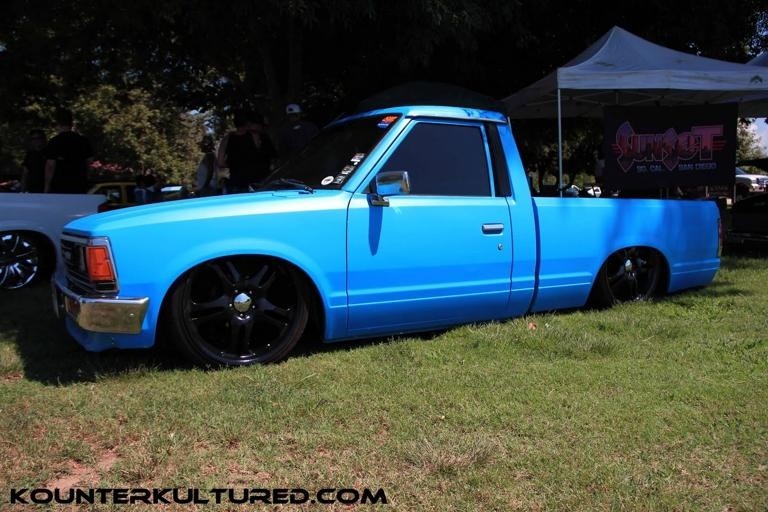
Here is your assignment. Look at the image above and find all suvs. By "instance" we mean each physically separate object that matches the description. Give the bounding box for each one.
[732,165,767,200]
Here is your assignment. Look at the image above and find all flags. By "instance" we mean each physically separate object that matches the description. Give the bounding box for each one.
[597,101,739,190]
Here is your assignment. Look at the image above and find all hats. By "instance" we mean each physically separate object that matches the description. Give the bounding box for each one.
[286,104,300,113]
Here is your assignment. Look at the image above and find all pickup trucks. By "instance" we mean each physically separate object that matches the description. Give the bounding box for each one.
[0,190,112,295]
[79,181,182,214]
[50,103,726,372]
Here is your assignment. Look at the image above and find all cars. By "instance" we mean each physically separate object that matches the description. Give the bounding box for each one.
[718,189,767,262]
[556,181,582,197]
[580,183,619,199]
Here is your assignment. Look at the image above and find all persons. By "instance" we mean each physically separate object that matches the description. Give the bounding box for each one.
[132,101,319,204]
[18,127,48,194]
[44,106,96,192]
